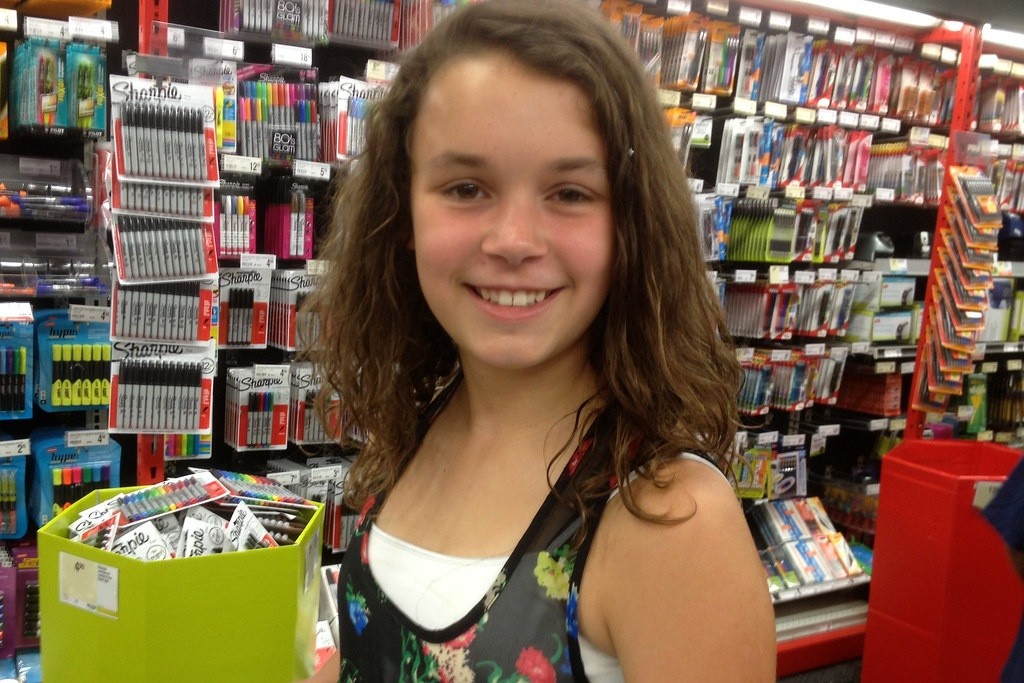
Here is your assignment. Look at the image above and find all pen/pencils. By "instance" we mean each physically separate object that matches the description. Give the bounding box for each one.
[603,0,1024,597]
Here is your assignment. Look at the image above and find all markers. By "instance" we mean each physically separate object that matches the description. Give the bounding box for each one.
[0,0,454,683]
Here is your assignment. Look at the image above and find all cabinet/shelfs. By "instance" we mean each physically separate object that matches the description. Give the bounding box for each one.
[838,259,1024,358]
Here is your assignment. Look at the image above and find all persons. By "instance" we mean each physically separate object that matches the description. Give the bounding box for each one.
[291,0,776,683]
